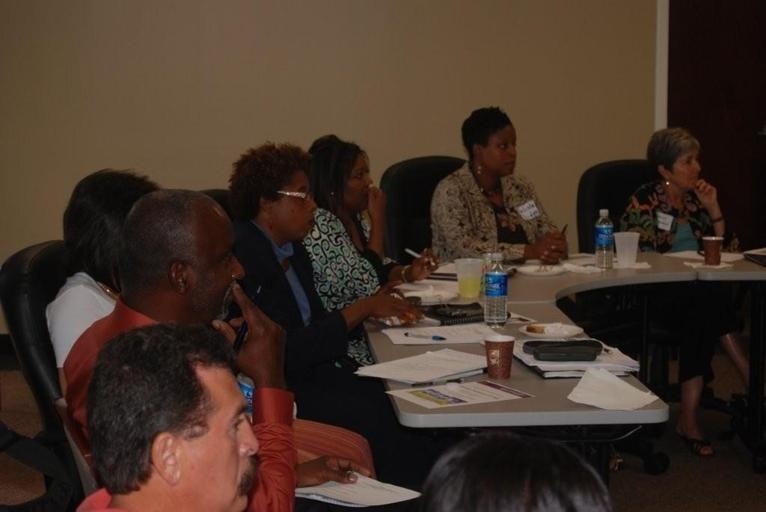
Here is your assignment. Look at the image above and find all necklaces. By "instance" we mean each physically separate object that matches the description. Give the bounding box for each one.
[482,185,502,196]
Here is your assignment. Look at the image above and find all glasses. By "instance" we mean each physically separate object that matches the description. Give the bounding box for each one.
[279,190,313,203]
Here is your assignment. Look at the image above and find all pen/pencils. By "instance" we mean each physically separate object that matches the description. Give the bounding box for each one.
[232,320,247,351]
[404,248,436,266]
[411,379,464,386]
[404,331,446,340]
[561,224,568,234]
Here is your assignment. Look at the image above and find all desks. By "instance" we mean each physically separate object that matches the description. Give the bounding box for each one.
[664,246,766,475]
[357,301,671,483]
[394,244,698,304]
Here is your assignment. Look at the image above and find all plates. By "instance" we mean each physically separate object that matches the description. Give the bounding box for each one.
[518,323,583,339]
[517,264,564,276]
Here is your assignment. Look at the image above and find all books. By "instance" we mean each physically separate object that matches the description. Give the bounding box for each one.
[514,335,640,381]
[352,346,488,388]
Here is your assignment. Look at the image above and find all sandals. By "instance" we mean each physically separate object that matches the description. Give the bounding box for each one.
[680,433,713,457]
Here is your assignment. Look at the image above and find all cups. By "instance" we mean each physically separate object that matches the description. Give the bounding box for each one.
[614,232,641,266]
[484,335,515,380]
[702,236,724,266]
[455,259,484,298]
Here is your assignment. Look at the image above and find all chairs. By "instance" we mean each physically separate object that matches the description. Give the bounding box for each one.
[1,239,99,512]
[577,158,744,475]
[378,154,468,265]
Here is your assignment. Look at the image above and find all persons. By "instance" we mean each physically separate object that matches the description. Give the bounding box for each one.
[422,429,612,512]
[301,135,440,367]
[429,106,571,265]
[228,143,425,428]
[75,320,261,512]
[59,187,374,511]
[44,170,161,371]
[619,125,751,456]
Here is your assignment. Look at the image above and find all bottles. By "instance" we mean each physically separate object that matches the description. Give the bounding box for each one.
[484,252,508,322]
[594,209,614,268]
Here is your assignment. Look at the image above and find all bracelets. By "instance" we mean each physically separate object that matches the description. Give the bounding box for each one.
[711,215,724,224]
